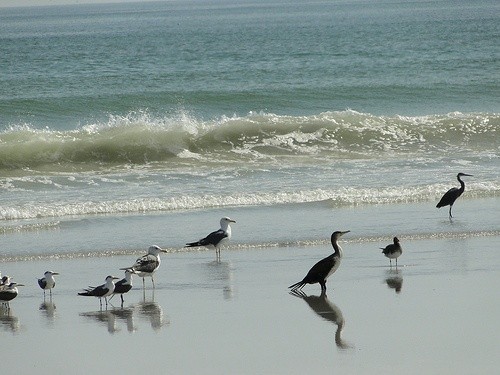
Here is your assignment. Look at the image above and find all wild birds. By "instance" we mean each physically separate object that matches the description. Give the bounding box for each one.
[120,245,168,288]
[38,271,60,294]
[184,217,236,252]
[379,236,403,265]
[0,275,25,314]
[436,172,473,218]
[288,230,351,289]
[78,267,136,308]
